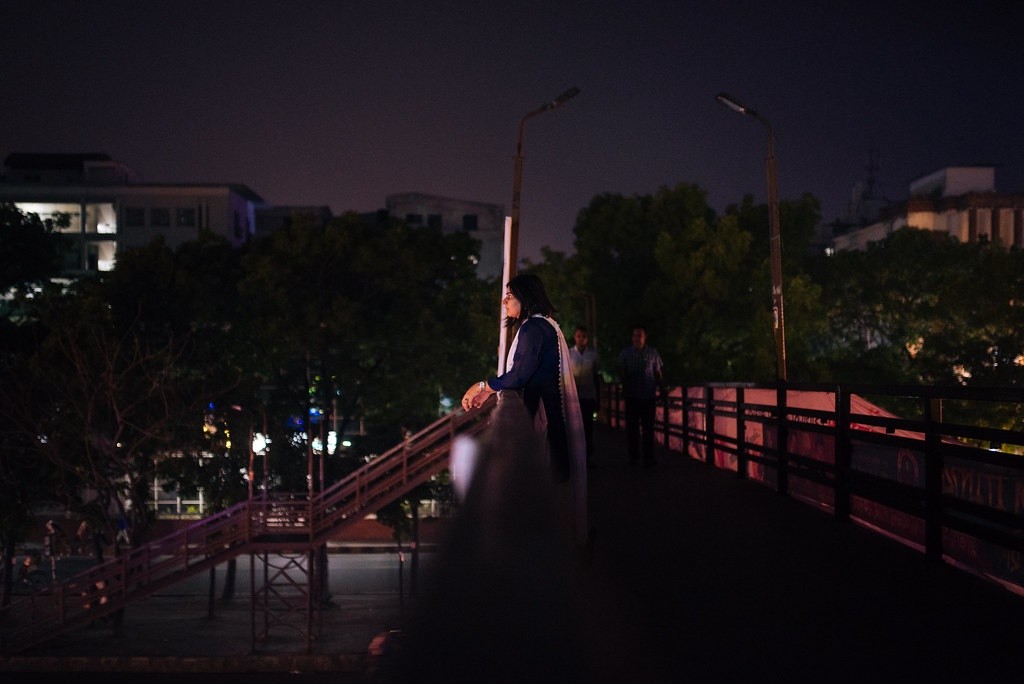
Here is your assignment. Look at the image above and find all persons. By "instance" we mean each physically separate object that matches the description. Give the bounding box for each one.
[459,276,589,634]
[566,326,600,425]
[611,326,666,459]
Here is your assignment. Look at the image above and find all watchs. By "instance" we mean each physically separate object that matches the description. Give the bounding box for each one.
[479,380,486,390]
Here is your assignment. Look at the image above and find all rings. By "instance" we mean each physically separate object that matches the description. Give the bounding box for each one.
[465,399,468,401]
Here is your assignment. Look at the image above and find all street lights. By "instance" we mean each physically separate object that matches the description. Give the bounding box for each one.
[504,83,582,379]
[229,389,460,601]
[712,93,794,494]
